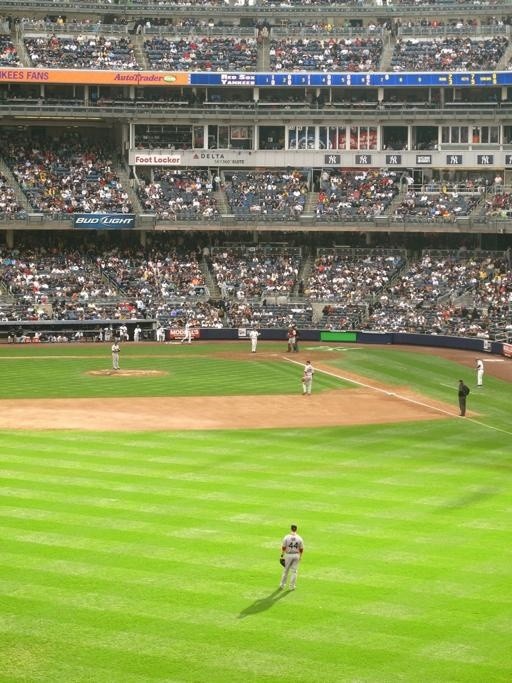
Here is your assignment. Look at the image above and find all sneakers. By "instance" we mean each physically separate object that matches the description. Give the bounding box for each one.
[303,392,312,396]
[279,584,297,591]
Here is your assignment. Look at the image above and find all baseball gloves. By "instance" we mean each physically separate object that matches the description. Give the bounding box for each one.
[280,559,285,566]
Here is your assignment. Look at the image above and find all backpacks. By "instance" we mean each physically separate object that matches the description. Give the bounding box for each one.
[463,385,470,397]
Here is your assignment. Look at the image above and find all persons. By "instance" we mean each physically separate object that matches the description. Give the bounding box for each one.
[303,360,314,395]
[475,356,485,386]
[111,340,121,370]
[0,0,512,74]
[279,525,304,590]
[1,80,511,223]
[458,379,470,416]
[17,228,511,354]
